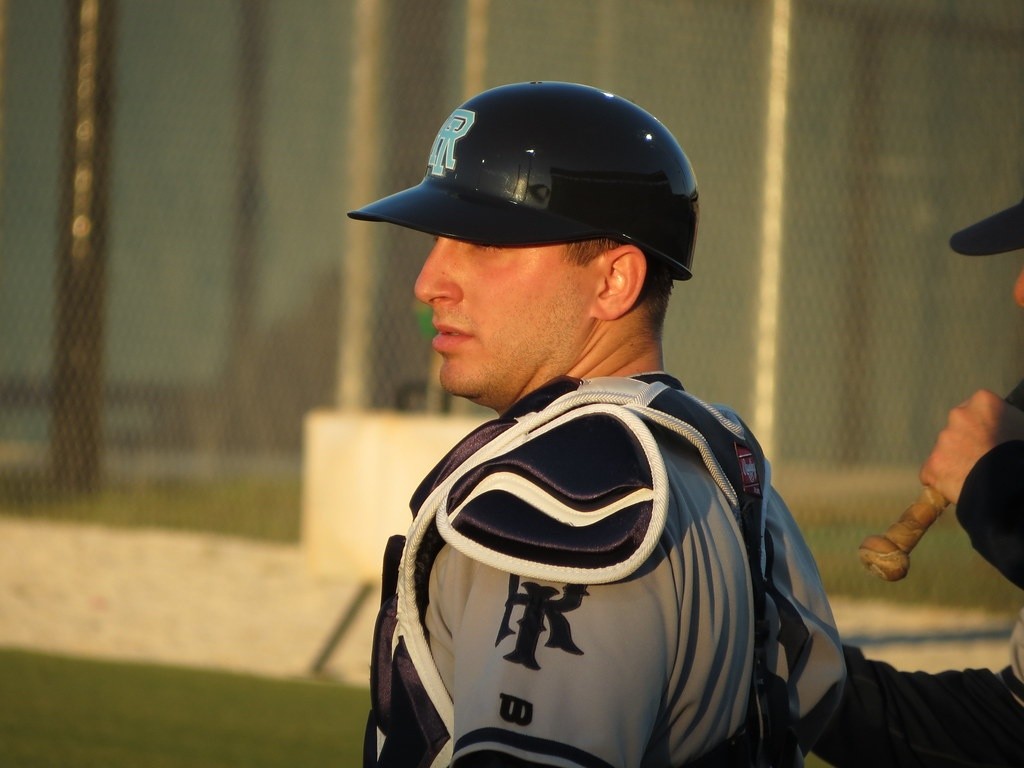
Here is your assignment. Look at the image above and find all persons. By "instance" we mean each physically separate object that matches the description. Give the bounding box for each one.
[347,82,846,768]
[814,200,1024,768]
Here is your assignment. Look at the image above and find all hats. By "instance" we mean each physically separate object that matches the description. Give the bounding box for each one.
[950,204,1024,257]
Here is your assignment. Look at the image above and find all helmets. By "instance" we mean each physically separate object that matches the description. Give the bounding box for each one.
[347,81,695,281]
